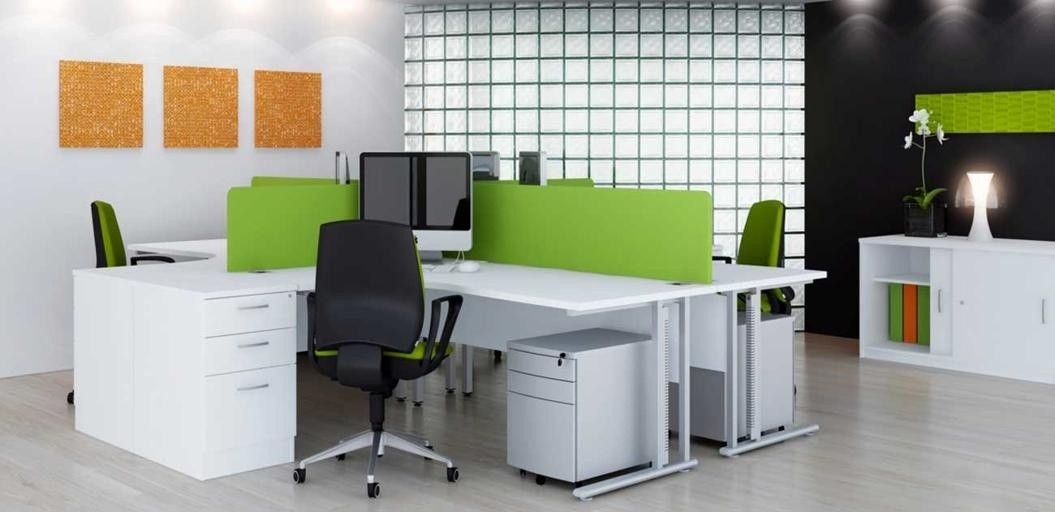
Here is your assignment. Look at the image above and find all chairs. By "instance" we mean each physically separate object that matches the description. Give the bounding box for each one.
[66,200,177,406]
[712,200,797,398]
[291,217,464,494]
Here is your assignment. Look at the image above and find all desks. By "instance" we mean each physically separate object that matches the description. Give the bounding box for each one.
[74,178,830,501]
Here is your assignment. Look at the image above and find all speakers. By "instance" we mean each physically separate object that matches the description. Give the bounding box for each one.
[519,152,540,185]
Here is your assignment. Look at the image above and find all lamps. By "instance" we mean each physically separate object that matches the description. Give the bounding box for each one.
[955,171,999,242]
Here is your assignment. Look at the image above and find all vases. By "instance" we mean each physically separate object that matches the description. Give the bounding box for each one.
[904,203,945,237]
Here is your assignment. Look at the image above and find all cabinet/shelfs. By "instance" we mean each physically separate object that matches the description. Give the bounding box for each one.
[930,238,1055,385]
[860,240,929,366]
[506,326,656,488]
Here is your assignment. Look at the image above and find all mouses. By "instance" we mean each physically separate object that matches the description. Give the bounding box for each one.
[458,262,480,272]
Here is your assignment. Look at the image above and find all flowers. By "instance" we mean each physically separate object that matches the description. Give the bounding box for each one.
[901,109,948,207]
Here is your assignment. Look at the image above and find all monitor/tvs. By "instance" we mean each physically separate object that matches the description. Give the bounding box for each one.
[466,150,500,181]
[358,152,473,260]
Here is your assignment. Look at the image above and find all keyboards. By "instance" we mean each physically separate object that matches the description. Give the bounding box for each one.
[418,264,455,274]
